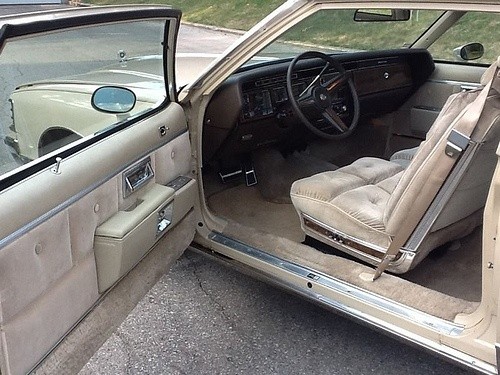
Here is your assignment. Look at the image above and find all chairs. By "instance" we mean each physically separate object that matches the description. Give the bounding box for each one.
[389,140,421,165]
[289,53,500,276]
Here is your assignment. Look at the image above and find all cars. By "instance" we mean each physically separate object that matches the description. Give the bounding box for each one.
[2,1,498,375]
